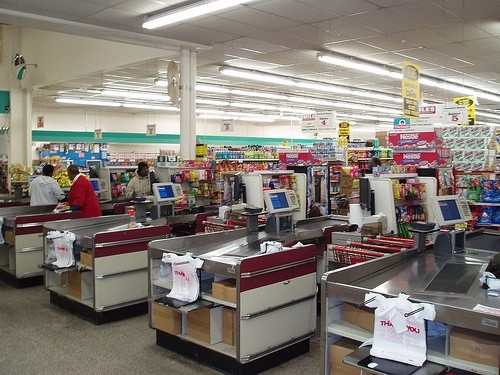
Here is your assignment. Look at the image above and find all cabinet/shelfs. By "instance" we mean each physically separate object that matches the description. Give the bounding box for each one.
[105,124,500,236]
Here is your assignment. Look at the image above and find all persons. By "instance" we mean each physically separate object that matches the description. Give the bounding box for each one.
[29,164,66,206]
[370,156,381,167]
[125,162,153,212]
[59,164,102,218]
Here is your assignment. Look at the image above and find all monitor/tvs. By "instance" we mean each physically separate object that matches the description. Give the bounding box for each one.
[270,191,289,209]
[233,173,247,204]
[91,181,99,192]
[158,185,175,198]
[359,178,376,216]
[438,199,461,221]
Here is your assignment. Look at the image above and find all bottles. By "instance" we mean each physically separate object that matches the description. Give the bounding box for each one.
[128,209,136,229]
[216,151,264,171]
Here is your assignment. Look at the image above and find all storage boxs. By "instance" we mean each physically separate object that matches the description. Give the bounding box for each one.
[5,229,500,375]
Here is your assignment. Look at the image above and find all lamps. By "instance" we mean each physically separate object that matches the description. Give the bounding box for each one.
[53,0,499,125]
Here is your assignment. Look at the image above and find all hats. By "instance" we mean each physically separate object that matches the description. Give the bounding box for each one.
[137,162,149,172]
